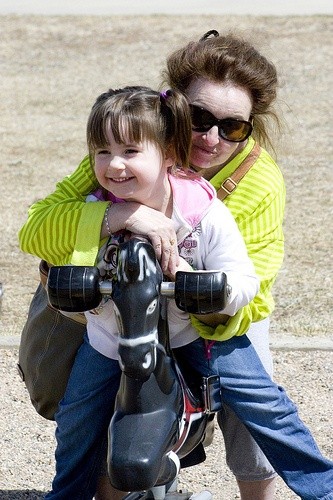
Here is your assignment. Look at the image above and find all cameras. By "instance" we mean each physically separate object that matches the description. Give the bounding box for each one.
[200,375,222,414]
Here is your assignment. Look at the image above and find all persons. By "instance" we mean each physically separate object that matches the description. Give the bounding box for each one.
[17,29,287,500]
[53,85,333,500]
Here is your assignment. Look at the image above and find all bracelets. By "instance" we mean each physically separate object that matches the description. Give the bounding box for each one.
[105,201,114,236]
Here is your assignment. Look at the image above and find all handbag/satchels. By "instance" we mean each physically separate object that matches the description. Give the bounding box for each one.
[15,259,88,421]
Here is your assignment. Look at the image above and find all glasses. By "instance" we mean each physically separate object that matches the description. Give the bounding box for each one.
[188,103,255,143]
